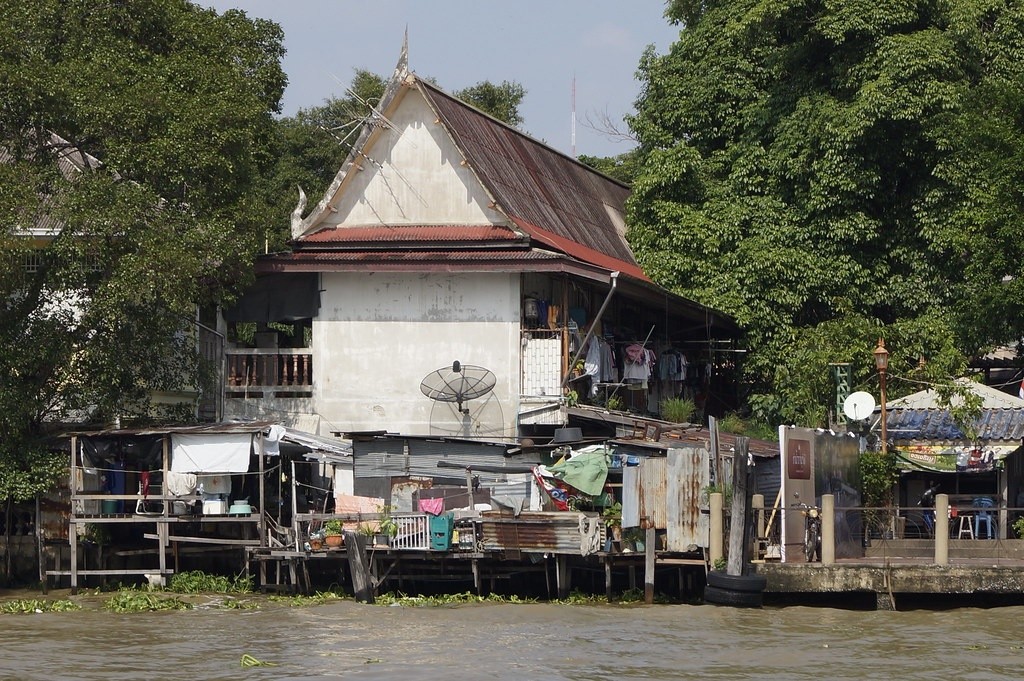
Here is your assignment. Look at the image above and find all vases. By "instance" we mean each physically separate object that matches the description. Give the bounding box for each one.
[309,539,322,550]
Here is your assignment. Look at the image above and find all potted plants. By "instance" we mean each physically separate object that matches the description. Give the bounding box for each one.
[624,527,646,552]
[375,504,399,545]
[324,517,344,548]
[361,520,379,545]
[603,502,623,540]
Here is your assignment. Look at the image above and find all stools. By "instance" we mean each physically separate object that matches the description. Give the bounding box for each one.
[957,516,974,540]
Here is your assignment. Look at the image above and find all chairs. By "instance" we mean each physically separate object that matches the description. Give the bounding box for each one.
[972,497,996,538]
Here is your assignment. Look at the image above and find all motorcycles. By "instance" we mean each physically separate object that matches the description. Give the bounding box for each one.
[790,492,823,562]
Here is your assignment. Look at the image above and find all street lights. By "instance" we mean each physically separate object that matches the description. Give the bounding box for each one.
[873,336,893,538]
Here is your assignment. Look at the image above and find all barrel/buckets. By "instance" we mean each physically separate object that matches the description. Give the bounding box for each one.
[103,499,118,514]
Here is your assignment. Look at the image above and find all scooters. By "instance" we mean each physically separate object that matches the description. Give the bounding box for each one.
[918,484,953,537]
